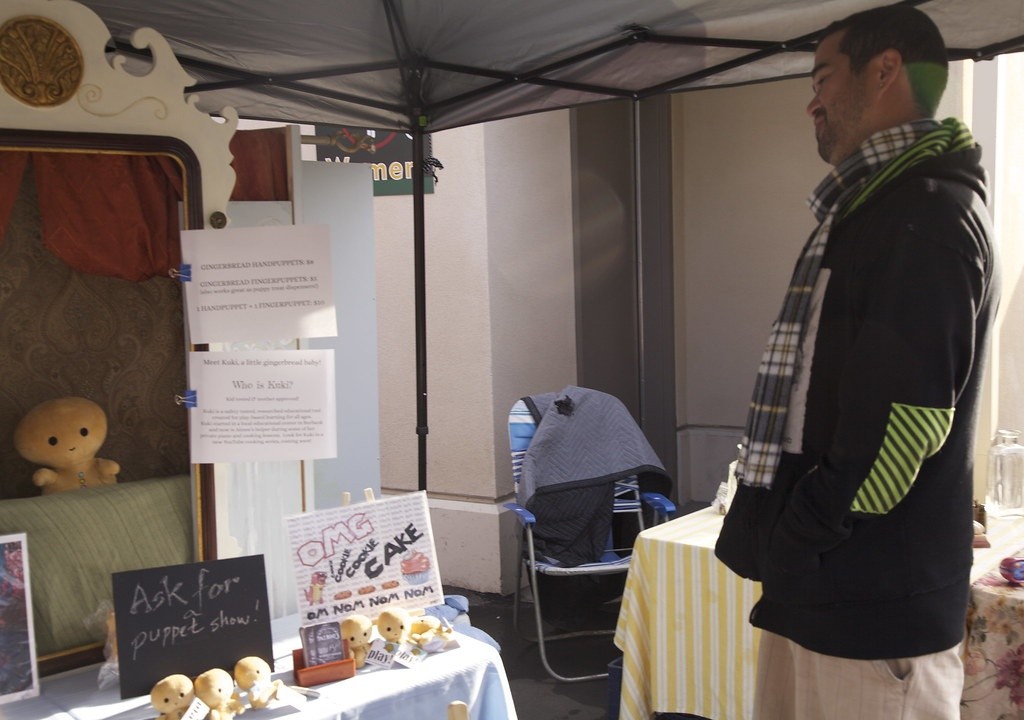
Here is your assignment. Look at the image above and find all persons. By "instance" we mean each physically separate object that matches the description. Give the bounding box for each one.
[712,4,1004,720]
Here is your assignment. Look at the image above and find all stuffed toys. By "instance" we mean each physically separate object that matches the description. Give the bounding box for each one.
[339,614,379,670]
[14,397,122,497]
[233,656,284,711]
[377,605,411,663]
[150,674,204,719]
[193,668,246,720]
[406,612,453,654]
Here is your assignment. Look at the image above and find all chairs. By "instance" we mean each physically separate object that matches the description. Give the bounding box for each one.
[501,386,676,682]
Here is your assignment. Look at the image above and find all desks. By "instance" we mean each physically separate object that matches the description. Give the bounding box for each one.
[0,606,500,720]
[639,506,1024,610]
